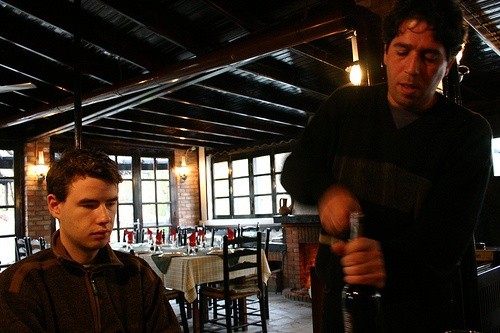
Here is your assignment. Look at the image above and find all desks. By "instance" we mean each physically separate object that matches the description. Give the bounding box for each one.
[135,248,271,333]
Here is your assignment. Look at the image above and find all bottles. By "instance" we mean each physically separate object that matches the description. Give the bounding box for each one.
[342,212,381,333]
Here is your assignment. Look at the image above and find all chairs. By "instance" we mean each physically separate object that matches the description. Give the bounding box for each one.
[14,236,46,262]
[123,223,272,333]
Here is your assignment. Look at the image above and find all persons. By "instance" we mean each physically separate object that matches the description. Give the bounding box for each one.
[0,147,184,333]
[281,0,492,333]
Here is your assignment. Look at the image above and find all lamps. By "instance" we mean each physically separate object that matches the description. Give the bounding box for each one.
[350,38,362,86]
[25,150,51,184]
[171,155,195,181]
